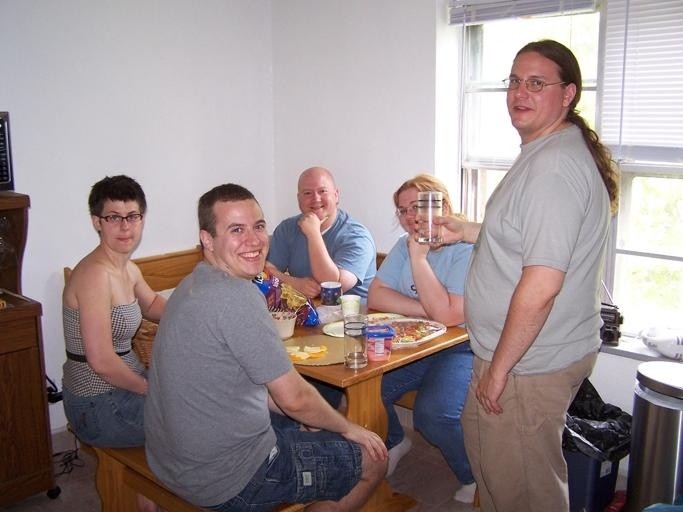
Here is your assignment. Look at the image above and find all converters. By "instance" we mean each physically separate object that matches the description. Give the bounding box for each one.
[48,391,63,403]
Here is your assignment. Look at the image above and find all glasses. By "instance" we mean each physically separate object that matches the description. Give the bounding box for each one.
[502,77,567,93]
[394,204,419,217]
[99,214,143,223]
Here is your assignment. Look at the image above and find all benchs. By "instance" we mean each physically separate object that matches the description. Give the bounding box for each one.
[61,240,205,301]
[65,414,432,512]
[374,252,421,410]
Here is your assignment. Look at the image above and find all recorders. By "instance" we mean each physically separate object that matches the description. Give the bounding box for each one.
[600,279,624,345]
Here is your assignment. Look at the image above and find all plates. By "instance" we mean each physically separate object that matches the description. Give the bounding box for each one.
[322,313,446,348]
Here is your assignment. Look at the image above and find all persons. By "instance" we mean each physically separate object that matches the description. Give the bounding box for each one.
[264,168,378,411]
[60,175,168,449]
[411,39,620,510]
[144,181,389,512]
[367,175,477,503]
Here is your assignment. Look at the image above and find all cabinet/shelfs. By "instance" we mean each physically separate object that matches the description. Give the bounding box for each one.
[0,188,62,511]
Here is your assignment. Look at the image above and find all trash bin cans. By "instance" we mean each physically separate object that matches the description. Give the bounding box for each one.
[562,397,632,512]
[624,360,683,512]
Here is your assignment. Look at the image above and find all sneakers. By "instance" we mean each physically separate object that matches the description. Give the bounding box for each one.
[450,481,479,505]
[384,438,412,479]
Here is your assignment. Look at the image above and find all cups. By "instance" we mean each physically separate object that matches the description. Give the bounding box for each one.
[340,295,363,316]
[342,313,368,368]
[319,283,342,306]
[417,191,443,245]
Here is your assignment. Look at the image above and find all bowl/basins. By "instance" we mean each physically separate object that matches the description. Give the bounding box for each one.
[268,312,295,339]
